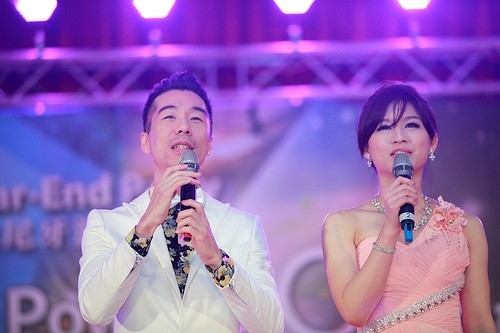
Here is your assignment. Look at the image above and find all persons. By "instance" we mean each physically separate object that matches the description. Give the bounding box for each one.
[320,80,498,333]
[78,73,284,333]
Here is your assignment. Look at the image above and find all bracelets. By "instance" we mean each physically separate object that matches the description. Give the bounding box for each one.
[372,243,397,254]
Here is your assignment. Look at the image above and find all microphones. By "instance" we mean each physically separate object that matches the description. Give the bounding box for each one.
[179,150,200,242]
[392,153,414,242]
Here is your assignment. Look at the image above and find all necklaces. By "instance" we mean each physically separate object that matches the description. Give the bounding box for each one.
[371,194,432,231]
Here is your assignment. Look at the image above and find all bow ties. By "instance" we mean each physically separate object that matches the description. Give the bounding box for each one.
[169,188,205,208]
